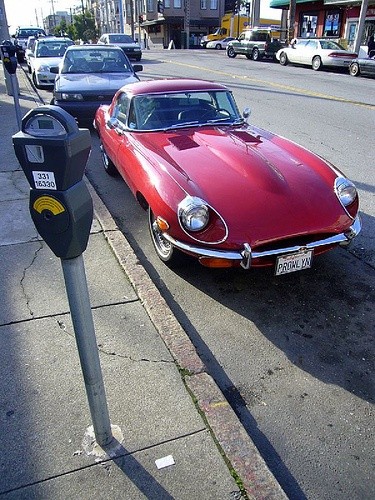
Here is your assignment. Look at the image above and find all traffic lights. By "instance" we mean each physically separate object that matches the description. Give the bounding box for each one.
[139,16,143,23]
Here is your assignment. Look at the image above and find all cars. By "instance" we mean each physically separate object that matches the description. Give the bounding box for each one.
[97,33,142,61]
[29,38,75,88]
[50,45,142,124]
[206,37,235,49]
[24,35,56,73]
[275,39,357,71]
[349,50,375,77]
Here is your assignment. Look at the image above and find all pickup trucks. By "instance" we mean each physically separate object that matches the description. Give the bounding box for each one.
[226,30,283,61]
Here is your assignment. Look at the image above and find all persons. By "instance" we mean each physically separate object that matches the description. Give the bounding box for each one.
[10,35,16,45]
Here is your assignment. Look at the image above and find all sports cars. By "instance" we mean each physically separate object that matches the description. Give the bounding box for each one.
[93,79,361,276]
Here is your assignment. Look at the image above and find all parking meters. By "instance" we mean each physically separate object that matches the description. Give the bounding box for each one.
[0,39,23,131]
[11,106,112,446]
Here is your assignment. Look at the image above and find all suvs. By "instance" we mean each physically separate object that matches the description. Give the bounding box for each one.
[12,28,47,64]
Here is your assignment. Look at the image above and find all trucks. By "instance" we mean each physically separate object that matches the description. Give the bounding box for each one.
[200,15,293,48]
